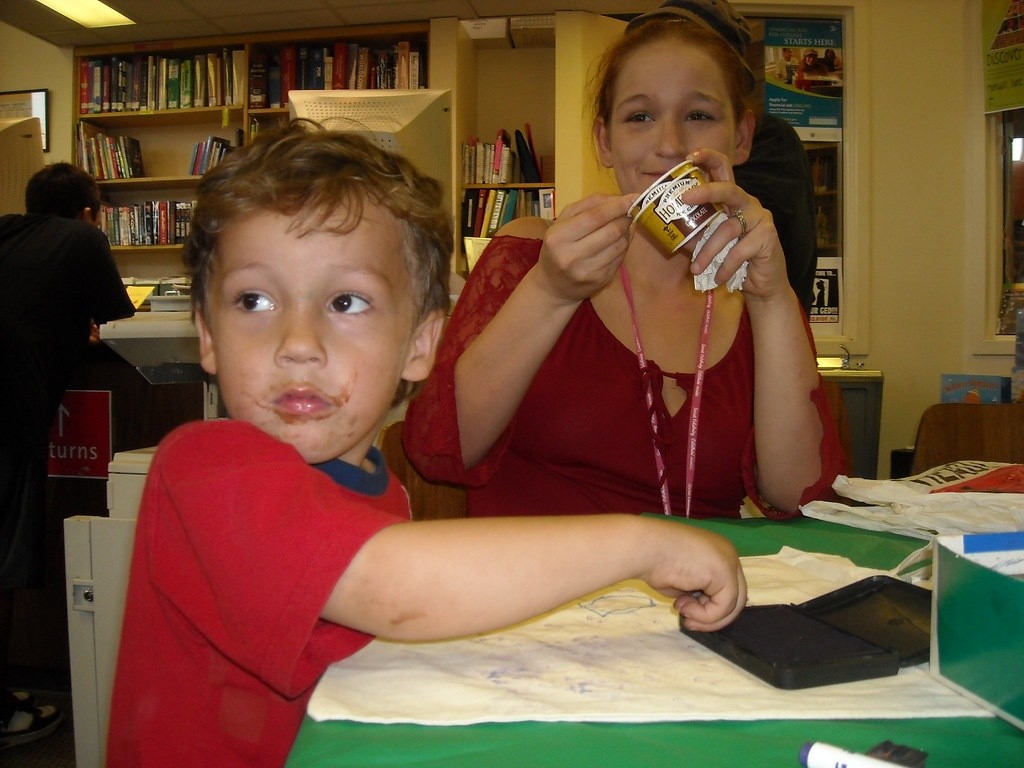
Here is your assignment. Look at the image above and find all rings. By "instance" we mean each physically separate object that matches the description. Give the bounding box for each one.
[729,207,748,238]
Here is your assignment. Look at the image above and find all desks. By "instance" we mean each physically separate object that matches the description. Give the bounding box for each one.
[275,515,1022,768]
[814,368,884,481]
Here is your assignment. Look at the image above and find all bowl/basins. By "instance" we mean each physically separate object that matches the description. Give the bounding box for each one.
[627,160,727,256]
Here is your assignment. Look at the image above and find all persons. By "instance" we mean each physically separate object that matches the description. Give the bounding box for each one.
[403,1,856,523]
[822,48,842,71]
[795,47,833,94]
[108,112,747,768]
[774,45,801,84]
[627,1,819,330]
[1,161,136,750]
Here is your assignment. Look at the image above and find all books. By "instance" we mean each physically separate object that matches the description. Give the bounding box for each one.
[74,119,146,181]
[247,39,428,110]
[79,46,246,115]
[95,199,199,246]
[188,116,262,178]
[461,119,555,273]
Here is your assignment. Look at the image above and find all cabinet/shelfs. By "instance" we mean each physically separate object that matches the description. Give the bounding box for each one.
[63,15,557,315]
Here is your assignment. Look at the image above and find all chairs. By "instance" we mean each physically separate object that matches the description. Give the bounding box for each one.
[908,403,1023,478]
